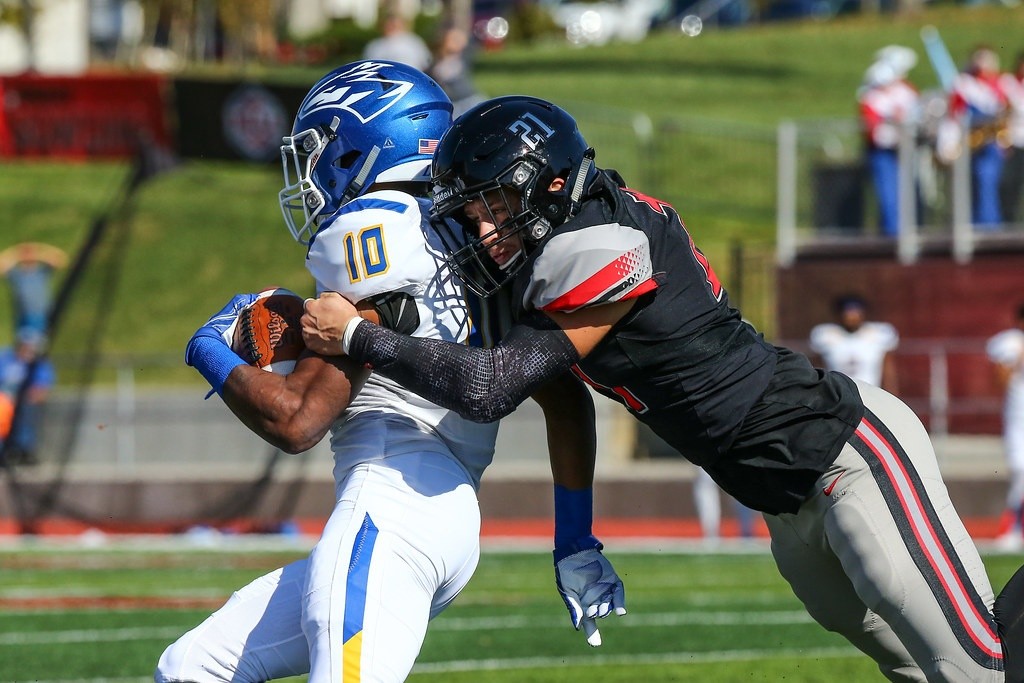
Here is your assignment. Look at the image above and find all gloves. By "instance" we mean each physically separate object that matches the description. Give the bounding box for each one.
[553,536,626,645]
[185,293,260,365]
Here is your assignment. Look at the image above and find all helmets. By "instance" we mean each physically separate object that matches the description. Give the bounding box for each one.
[279,60,453,246]
[428,95,596,299]
[833,290,869,315]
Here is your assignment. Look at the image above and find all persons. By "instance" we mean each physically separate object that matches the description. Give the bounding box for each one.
[300,97,1005,683]
[155,59,627,683]
[808,291,898,397]
[857,44,1024,234]
[988,302,1024,547]
[0,243,72,466]
[153,0,475,98]
[992,563,1024,683]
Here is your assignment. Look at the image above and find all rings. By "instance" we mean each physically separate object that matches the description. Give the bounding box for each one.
[303,298,314,309]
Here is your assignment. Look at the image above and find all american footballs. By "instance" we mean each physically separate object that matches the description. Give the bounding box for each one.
[232,285,305,375]
